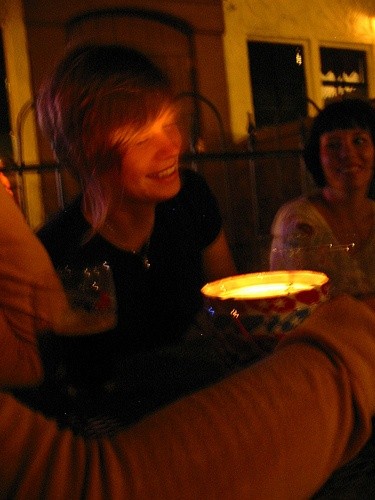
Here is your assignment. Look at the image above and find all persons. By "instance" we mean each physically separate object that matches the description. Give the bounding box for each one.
[33,33,238,334]
[0,293,375,499]
[2,161,72,391]
[269,91,374,310]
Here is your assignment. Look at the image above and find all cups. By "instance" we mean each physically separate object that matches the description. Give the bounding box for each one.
[29,266,120,438]
[201,269,332,383]
